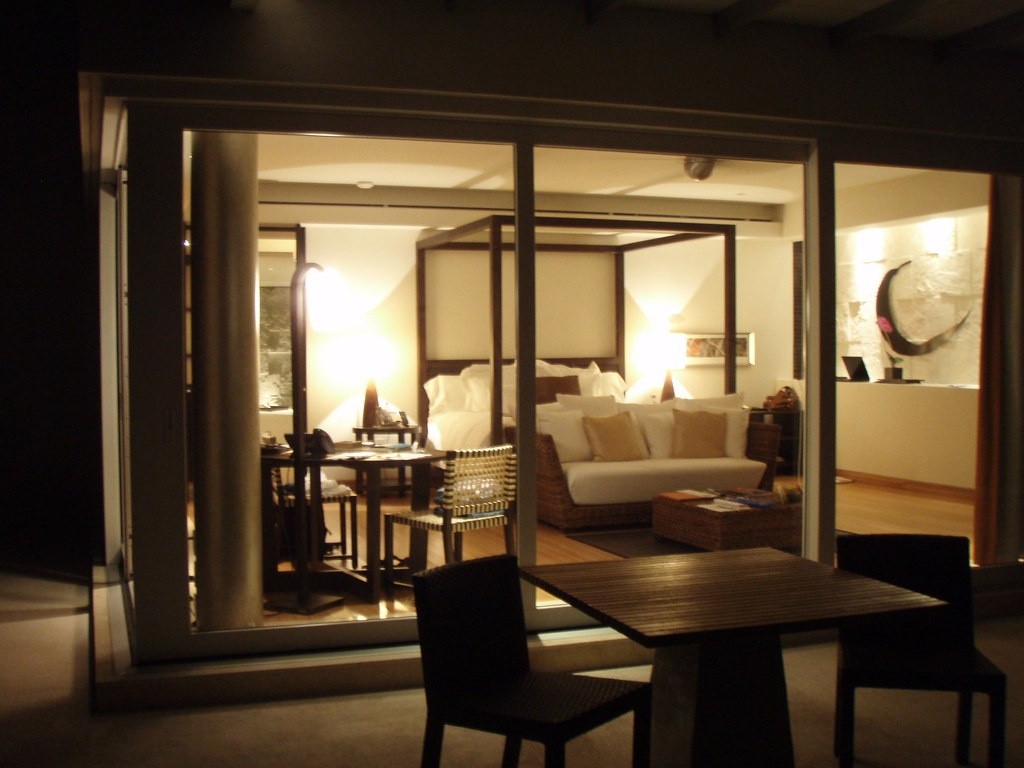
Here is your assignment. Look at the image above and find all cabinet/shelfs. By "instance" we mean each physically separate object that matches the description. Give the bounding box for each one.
[751,409,801,475]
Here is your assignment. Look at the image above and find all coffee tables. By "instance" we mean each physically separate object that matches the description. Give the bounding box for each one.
[654,487,802,553]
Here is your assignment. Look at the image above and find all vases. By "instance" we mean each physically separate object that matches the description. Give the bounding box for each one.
[884,366,902,380]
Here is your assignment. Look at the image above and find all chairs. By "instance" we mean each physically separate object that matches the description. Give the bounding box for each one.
[412,552,654,768]
[386,443,516,598]
[835,534,1007,768]
[268,438,359,569]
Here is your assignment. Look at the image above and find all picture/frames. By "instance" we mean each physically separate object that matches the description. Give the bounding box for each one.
[683,332,755,366]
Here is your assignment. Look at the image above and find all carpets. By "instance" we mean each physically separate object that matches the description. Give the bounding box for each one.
[566,529,853,560]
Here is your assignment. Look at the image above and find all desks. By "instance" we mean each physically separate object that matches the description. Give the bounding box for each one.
[261,442,449,604]
[353,427,423,500]
[519,545,949,768]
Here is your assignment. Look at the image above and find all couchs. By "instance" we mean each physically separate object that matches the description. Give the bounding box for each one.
[505,401,780,530]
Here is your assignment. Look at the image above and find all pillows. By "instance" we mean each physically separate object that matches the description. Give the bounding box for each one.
[423,358,626,414]
[538,391,752,463]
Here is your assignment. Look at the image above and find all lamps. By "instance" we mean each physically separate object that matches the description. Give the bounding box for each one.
[685,156,717,182]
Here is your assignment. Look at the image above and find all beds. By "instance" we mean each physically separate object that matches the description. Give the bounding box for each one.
[421,358,715,507]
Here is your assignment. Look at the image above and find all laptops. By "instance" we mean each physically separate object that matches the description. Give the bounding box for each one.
[842,356,870,382]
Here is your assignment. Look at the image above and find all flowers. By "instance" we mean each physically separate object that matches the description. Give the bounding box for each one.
[876,317,904,367]
